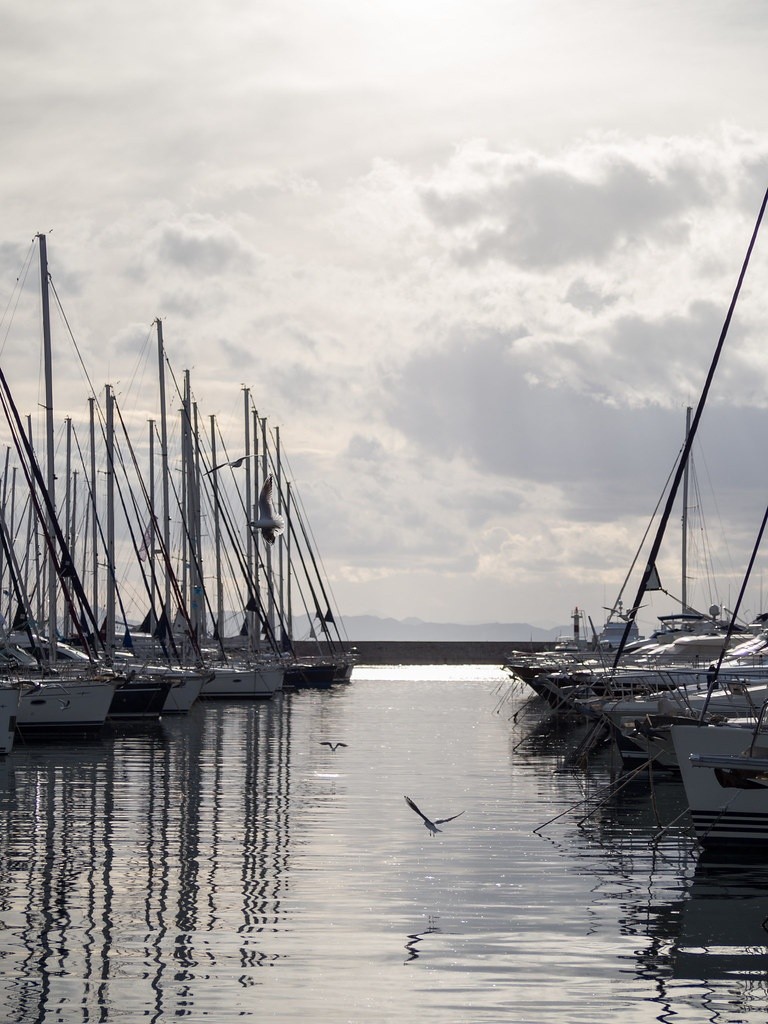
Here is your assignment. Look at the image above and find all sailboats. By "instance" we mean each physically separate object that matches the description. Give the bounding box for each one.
[0,222,358,756]
[501,402,768,855]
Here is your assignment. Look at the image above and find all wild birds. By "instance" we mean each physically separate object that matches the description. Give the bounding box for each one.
[201,454,263,477]
[319,741,349,755]
[403,795,465,837]
[244,472,285,547]
